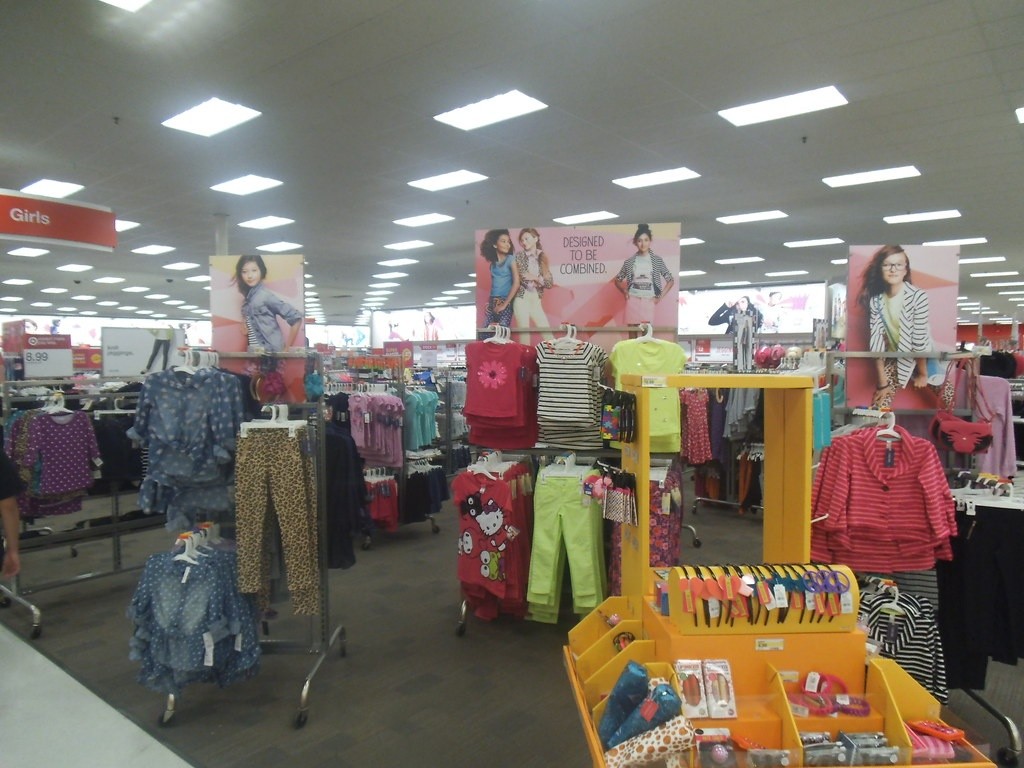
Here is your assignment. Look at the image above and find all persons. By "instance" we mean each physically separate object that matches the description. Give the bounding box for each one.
[0,446,23,579]
[614,223,675,339]
[140,328,173,374]
[423,312,439,340]
[759,291,784,333]
[389,322,410,341]
[343,333,354,346]
[168,321,205,346]
[477,229,521,341]
[854,245,955,410]
[513,228,555,346]
[229,255,303,377]
[25,318,61,335]
[708,296,764,335]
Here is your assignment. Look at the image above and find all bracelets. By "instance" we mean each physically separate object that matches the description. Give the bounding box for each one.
[786,673,869,718]
[876,381,890,390]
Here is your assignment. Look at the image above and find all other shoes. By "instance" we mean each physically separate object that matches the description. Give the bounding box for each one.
[141,368,150,374]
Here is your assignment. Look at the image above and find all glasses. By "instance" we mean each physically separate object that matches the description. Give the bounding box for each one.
[881,262,907,270]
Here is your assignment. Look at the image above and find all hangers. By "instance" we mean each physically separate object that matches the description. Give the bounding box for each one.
[831,408,900,445]
[737,442,764,462]
[775,351,845,394]
[864,575,906,615]
[35,350,308,566]
[949,471,1024,516]
[324,380,442,484]
[467,323,669,489]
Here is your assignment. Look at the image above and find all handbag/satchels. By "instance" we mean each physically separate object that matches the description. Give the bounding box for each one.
[928,355,996,455]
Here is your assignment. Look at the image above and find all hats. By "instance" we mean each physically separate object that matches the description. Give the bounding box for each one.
[753,345,801,367]
